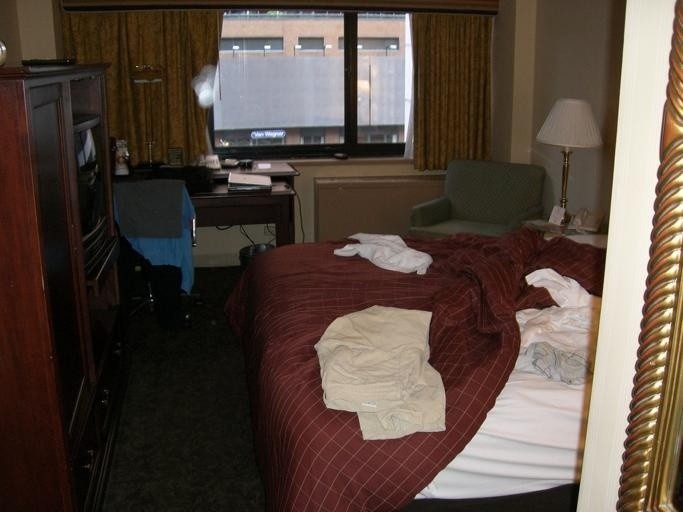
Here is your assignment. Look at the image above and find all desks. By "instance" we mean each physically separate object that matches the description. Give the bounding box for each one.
[113,162,300,247]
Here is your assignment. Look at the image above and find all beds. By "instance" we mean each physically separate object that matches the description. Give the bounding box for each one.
[248,233,607,510]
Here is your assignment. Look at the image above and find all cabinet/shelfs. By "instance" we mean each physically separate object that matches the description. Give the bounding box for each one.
[0,62,131,509]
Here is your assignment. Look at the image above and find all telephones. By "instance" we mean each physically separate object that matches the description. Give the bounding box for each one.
[573,207,606,232]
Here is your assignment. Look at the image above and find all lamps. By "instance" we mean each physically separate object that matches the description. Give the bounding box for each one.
[536,97,605,228]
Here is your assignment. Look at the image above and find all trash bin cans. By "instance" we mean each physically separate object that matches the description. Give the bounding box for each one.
[240,243,275,272]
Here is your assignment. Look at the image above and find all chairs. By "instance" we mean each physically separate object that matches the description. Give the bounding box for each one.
[114,180,202,320]
[408,160,547,243]
